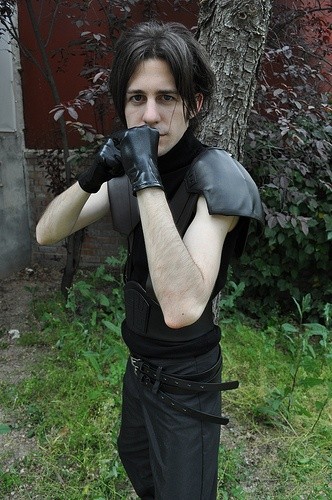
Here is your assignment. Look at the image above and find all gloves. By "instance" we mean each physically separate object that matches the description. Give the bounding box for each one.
[112,124,167,197]
[78,136,125,194]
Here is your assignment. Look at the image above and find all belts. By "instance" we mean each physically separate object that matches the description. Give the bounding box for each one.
[127,343,239,425]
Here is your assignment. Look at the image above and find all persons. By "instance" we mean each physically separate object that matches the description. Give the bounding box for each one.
[35,19,262,500]
